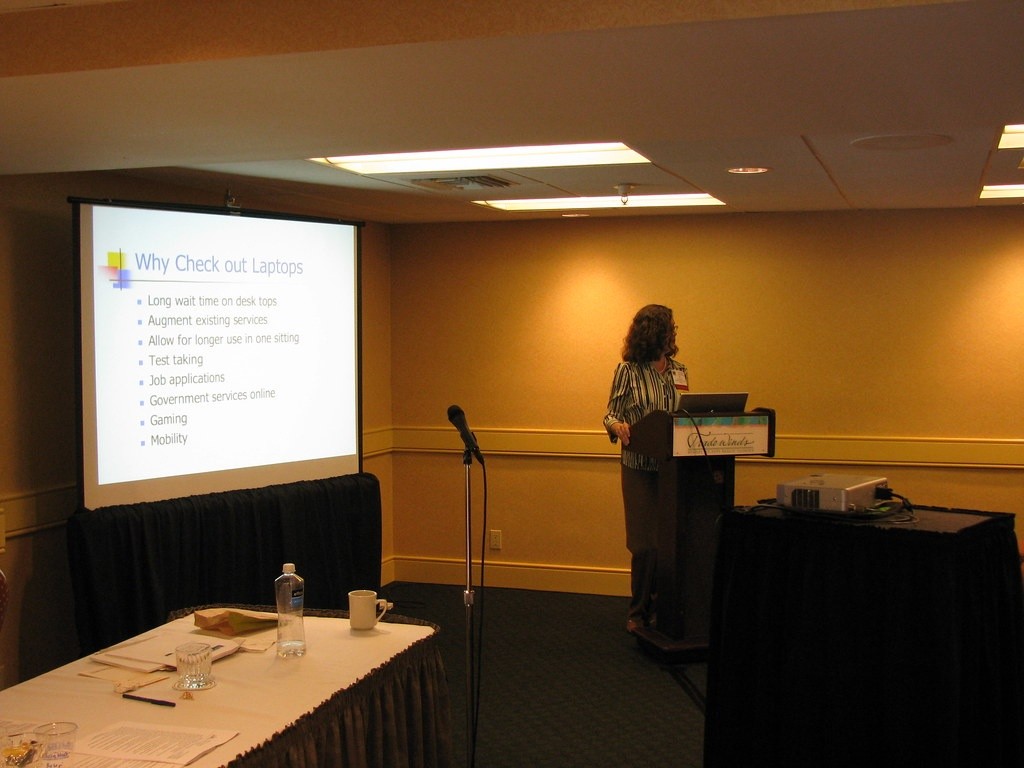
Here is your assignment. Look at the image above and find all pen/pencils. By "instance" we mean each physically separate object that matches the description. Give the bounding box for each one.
[123,693,176,708]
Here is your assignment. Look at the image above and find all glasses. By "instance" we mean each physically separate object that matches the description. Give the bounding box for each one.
[665,325,678,335]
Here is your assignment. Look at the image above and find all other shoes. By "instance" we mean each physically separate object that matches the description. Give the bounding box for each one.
[627,619,636,632]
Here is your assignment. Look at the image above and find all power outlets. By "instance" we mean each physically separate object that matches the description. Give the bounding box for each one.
[490,530,502,549]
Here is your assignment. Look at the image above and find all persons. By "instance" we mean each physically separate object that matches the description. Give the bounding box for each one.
[602,304,689,629]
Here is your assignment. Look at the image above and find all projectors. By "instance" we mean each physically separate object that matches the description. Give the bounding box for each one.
[776,473,888,512]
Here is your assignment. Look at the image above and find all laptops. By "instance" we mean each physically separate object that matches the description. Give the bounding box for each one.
[676,392,750,414]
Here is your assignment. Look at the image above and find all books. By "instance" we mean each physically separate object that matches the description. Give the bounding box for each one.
[90,631,246,673]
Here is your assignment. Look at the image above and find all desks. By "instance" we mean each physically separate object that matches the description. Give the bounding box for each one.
[704,503,1024,768]
[0,602,452,768]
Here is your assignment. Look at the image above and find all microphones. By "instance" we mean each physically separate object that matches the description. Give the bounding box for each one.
[447,405,485,465]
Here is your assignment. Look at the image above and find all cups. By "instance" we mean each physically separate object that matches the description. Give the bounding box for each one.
[33,721,77,768]
[348,590,387,630]
[175,643,212,684]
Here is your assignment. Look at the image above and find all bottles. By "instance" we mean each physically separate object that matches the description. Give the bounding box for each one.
[275,563,305,658]
